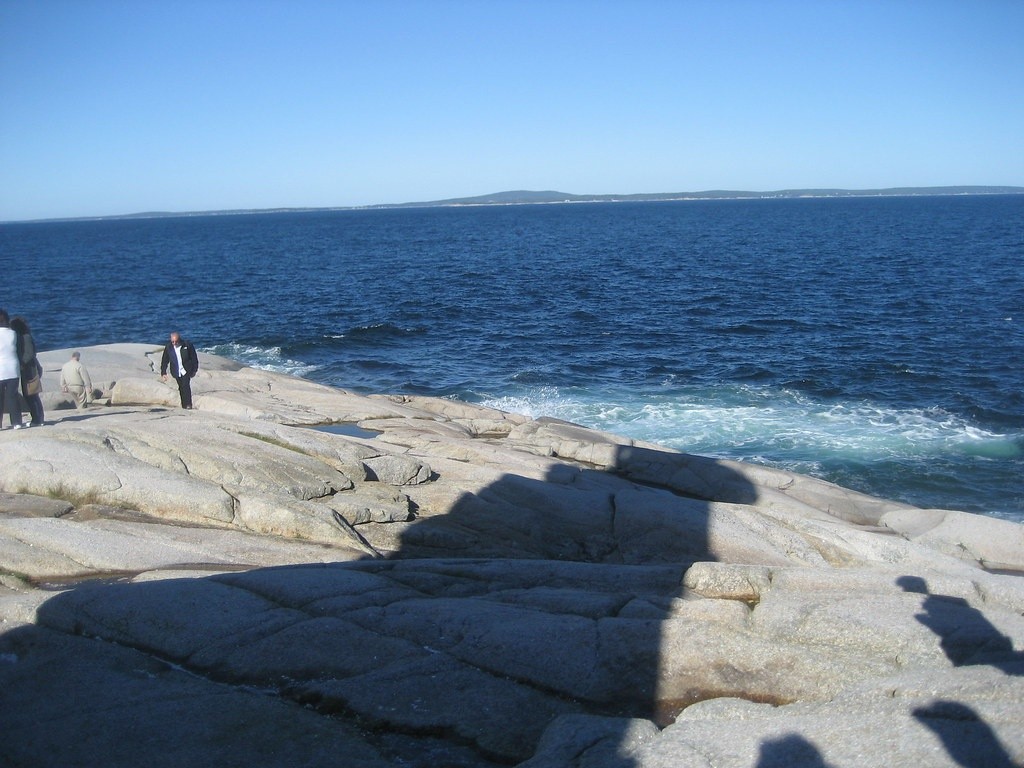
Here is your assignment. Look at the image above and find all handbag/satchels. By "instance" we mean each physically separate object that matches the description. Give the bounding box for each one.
[26,375,42,396]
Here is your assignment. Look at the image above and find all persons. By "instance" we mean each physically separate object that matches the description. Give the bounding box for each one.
[60,352,92,409]
[0,311,23,430]
[161,333,198,410]
[10,318,44,427]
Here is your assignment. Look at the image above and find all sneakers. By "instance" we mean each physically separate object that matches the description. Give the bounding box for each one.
[0,428,2,431]
[26,421,45,428]
[13,425,22,430]
